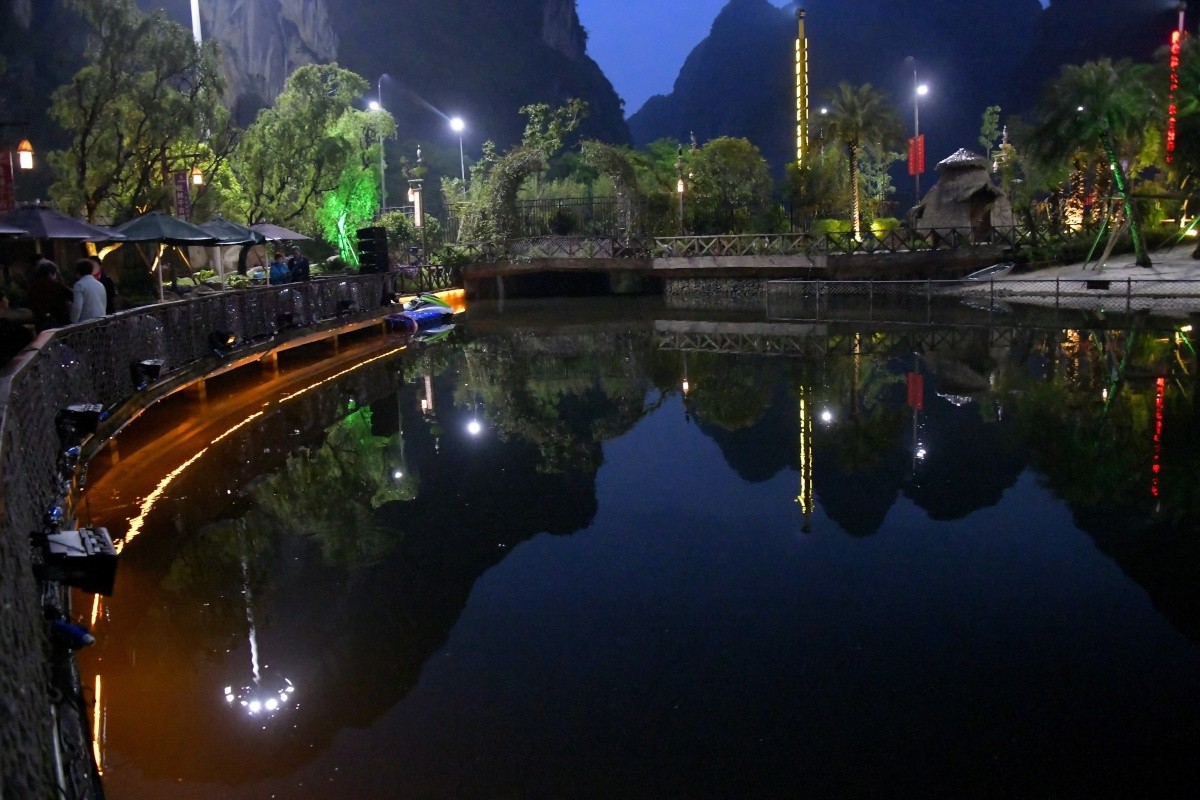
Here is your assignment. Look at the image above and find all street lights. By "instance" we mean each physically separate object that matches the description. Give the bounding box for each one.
[376,73,386,209]
[674,144,689,235]
[450,116,471,200]
[912,70,928,205]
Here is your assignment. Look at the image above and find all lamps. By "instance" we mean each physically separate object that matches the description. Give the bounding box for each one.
[138,358,165,381]
[55,403,105,435]
[343,300,358,311]
[215,328,236,347]
[28,525,117,599]
[283,312,300,324]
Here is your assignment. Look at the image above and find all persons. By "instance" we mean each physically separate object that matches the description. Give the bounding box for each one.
[288,246,310,282]
[29,256,116,337]
[270,254,293,286]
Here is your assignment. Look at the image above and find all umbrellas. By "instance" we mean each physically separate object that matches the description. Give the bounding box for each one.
[194,217,267,296]
[248,223,315,288]
[0,204,128,242]
[81,210,222,303]
